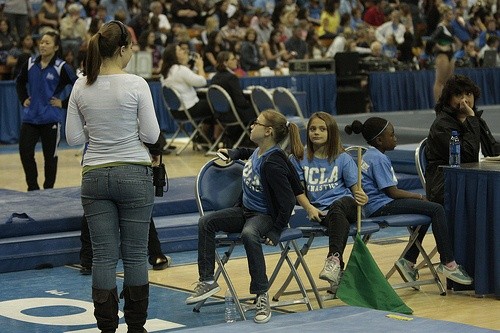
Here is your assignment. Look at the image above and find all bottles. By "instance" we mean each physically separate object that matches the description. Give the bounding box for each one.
[449,131,461,168]
[225,288,237,323]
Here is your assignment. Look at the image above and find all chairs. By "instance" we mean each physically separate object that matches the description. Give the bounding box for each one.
[346,147,446,296]
[250,85,274,117]
[415,138,428,188]
[192,157,312,321]
[161,85,212,155]
[207,84,250,155]
[272,86,307,146]
[272,201,380,308]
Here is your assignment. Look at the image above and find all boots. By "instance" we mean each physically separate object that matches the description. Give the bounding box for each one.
[120,282,150,333]
[91,285,121,333]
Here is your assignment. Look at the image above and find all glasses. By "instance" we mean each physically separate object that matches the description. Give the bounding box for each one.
[252,121,269,128]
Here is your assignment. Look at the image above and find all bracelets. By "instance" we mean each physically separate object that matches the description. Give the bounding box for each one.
[419,193,422,199]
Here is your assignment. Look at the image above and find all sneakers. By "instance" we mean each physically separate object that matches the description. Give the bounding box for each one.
[435,263,474,286]
[394,258,421,289]
[327,270,345,294]
[253,293,272,324]
[318,252,341,283]
[184,281,221,305]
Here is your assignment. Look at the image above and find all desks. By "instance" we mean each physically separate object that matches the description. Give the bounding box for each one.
[0,73,336,142]
[438,160,500,301]
[362,66,500,112]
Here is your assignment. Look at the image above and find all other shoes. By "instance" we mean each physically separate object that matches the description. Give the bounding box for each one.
[80,266,92,275]
[153,255,172,270]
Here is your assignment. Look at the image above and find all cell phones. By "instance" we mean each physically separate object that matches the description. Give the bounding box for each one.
[216,151,229,161]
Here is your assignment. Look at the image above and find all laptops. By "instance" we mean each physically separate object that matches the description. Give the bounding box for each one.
[482,50,497,67]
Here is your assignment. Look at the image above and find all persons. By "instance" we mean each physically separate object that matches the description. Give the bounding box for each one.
[0,0,500,81]
[185,109,325,324]
[344,117,474,290]
[289,111,368,283]
[428,4,457,104]
[211,51,258,147]
[162,42,222,143]
[15,31,77,191]
[79,134,172,276]
[64,20,161,333]
[425,74,500,207]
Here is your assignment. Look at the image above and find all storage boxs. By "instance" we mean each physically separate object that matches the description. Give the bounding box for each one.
[289,59,336,74]
